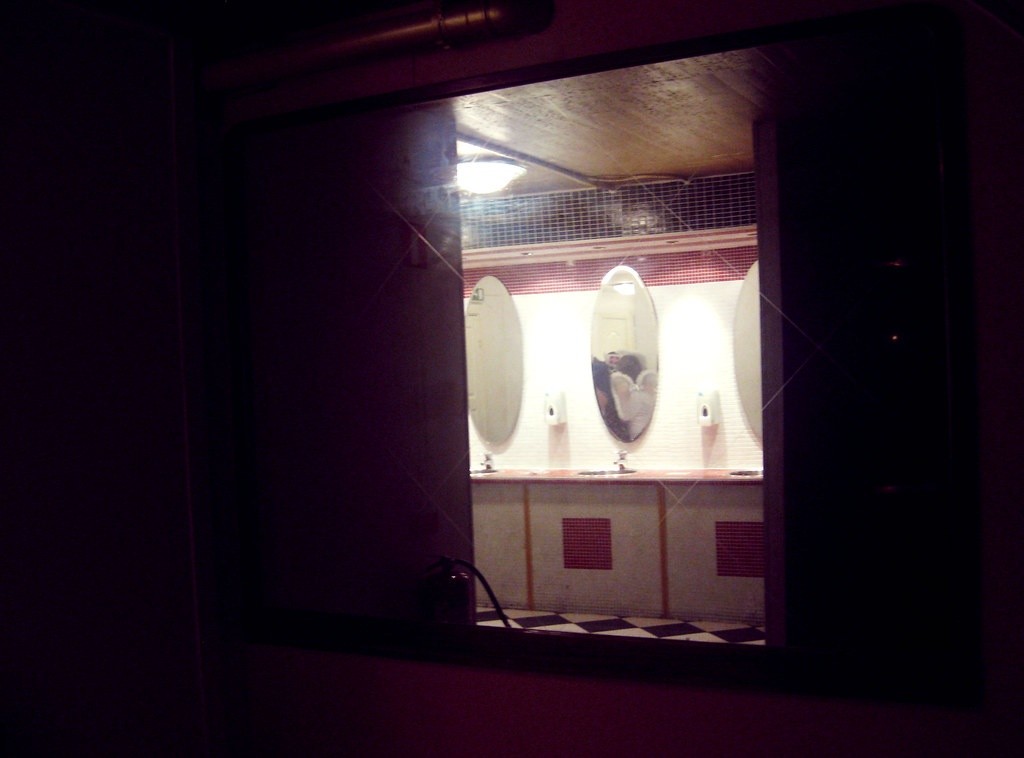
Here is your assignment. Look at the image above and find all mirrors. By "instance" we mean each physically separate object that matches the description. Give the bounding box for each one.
[589,266,660,444]
[464,276,525,446]
[734,261,764,446]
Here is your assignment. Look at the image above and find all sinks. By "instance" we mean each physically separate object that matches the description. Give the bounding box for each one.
[469,469,498,474]
[577,470,639,475]
[729,470,764,475]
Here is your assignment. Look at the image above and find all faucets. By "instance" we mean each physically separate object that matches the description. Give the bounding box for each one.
[613,450,628,471]
[480,451,494,469]
